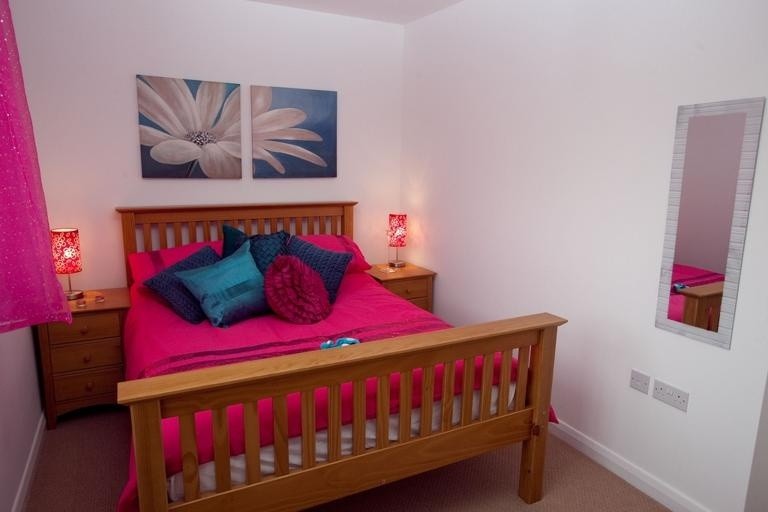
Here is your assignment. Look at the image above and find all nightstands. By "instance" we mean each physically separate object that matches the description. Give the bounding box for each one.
[362,259,437,316]
[36,287,129,428]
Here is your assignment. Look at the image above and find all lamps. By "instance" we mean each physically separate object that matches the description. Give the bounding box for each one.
[48,228,84,301]
[387,213,407,268]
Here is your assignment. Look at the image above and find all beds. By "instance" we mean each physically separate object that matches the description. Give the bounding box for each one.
[112,200,569,512]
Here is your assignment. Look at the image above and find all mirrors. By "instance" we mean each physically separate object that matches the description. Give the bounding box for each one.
[651,94,764,353]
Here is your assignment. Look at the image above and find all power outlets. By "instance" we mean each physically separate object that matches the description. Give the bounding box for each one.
[651,378,690,414]
[628,368,652,395]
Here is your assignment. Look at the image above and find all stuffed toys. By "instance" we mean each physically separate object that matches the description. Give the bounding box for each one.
[320,337,361,349]
[672,282,690,292]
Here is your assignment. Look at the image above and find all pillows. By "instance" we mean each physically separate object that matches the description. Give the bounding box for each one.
[220,222,291,275]
[174,238,271,329]
[264,253,333,326]
[284,233,355,305]
[142,244,222,324]
